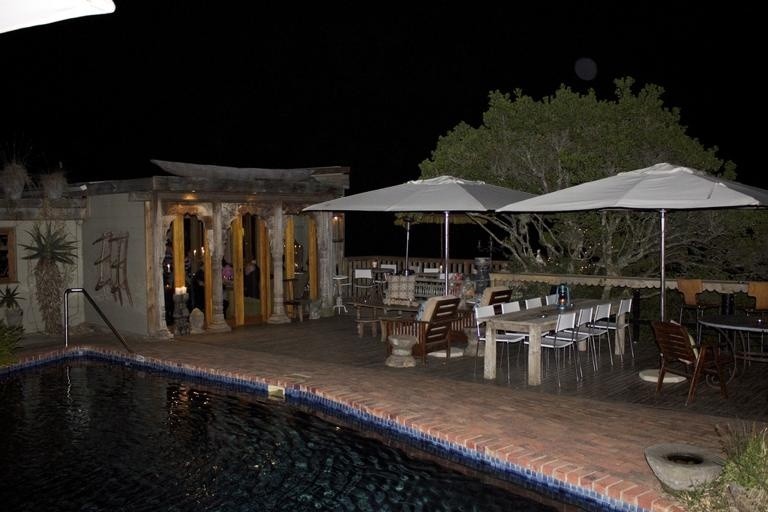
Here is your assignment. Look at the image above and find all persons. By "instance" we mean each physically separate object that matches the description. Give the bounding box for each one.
[192,261,260,319]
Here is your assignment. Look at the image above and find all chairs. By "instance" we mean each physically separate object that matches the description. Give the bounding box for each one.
[519,285,766,321]
[524,297,542,310]
[386,294,460,367]
[523,312,580,388]
[500,301,550,368]
[546,293,559,305]
[652,320,730,406]
[449,286,513,341]
[562,303,614,371]
[545,306,597,379]
[588,298,635,363]
[473,305,525,385]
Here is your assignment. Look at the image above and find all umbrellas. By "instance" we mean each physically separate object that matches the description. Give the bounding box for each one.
[302,174,542,295]
[493,161,767,322]
